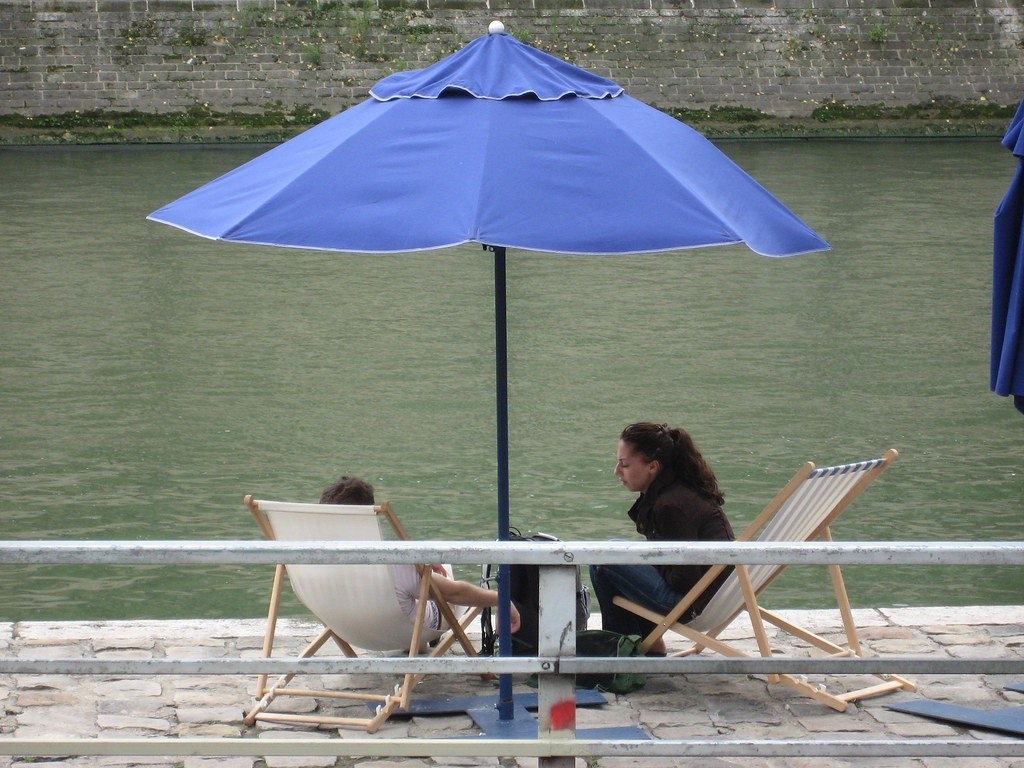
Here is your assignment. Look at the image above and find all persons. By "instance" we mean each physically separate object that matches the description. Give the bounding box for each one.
[319,476,520,655]
[589,422,736,658]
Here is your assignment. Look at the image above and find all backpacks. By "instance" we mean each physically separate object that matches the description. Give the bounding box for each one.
[480,528,593,653]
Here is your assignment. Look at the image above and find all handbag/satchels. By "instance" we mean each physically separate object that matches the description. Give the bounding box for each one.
[525,629,648,692]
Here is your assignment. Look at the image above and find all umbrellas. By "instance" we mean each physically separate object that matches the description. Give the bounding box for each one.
[146,20,834,721]
[990,97,1024,415]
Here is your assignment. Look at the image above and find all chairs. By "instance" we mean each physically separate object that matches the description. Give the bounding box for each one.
[242,494,490,734]
[613,449,917,713]
